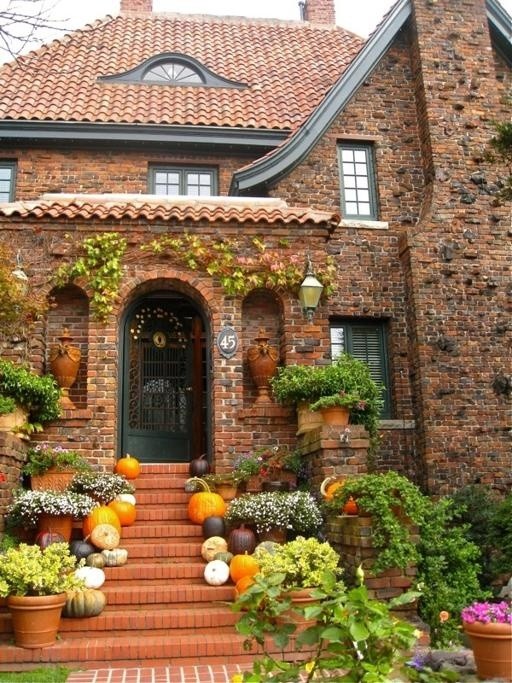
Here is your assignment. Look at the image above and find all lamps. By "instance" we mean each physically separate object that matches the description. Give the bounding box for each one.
[298,253,324,323]
[7,266,30,314]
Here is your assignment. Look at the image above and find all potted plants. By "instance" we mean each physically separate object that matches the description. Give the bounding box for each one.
[267,360,357,436]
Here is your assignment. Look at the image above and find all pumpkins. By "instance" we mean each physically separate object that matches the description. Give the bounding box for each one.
[187,451,269,612]
[38,454,141,619]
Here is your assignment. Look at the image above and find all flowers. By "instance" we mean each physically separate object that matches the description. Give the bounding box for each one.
[460,601,510,624]
[0,360,135,599]
[194,444,346,601]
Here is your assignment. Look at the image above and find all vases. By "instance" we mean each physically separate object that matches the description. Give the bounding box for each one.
[247,328,278,403]
[272,585,322,636]
[8,590,67,649]
[48,325,83,408]
[466,625,512,678]
